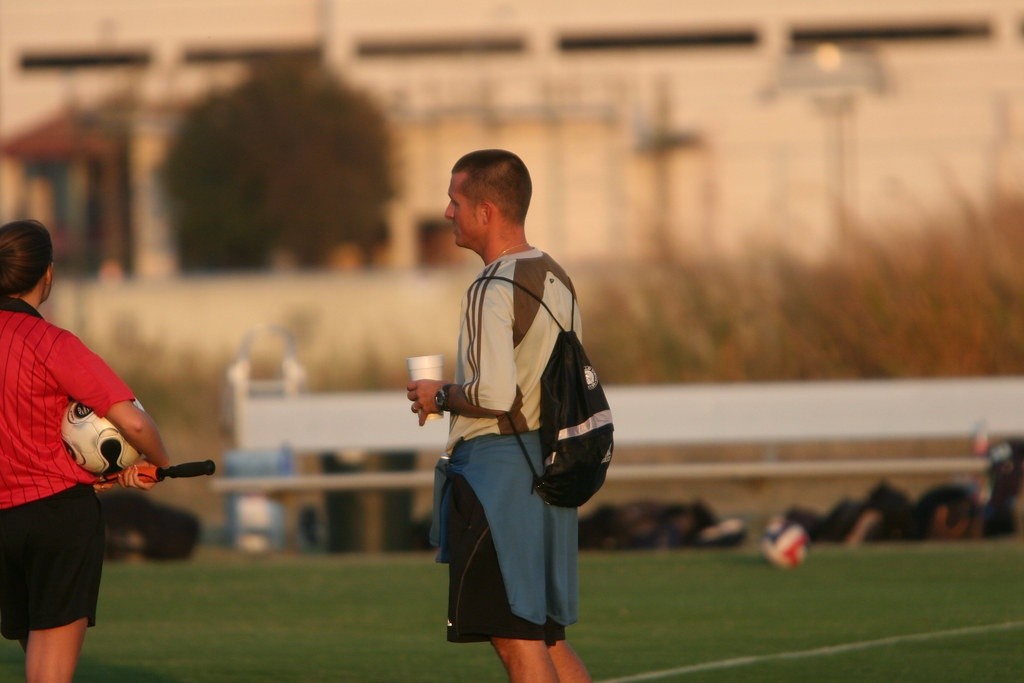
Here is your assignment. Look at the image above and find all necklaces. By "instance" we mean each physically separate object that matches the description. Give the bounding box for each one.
[496,242,530,259]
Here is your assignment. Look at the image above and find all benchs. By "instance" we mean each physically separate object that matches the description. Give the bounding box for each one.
[213,354,1024,553]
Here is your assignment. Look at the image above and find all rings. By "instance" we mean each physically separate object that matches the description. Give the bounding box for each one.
[412,403,420,411]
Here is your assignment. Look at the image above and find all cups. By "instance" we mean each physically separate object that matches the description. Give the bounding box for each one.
[406,354,444,420]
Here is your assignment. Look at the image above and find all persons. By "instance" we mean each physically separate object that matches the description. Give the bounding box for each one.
[406,148,594,683]
[0,219,174,683]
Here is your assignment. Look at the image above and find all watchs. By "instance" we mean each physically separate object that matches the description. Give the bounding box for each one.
[435,383,457,412]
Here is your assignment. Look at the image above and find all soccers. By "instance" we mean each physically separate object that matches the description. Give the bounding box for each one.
[759,516,810,567]
[60,397,145,478]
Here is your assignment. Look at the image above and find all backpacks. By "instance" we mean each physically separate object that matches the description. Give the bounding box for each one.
[468,277,613,506]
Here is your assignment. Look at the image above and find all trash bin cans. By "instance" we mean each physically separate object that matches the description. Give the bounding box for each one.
[320,449,418,557]
[223,442,291,553]
